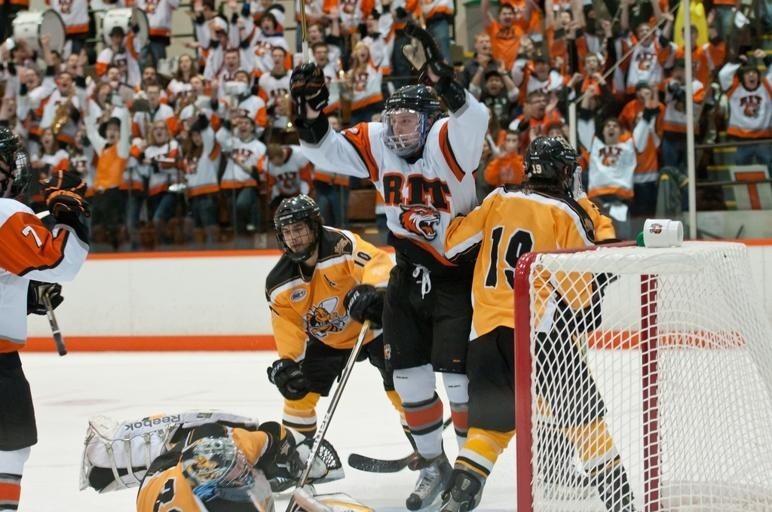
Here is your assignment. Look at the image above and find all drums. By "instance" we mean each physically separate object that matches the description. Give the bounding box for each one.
[104,6,151,47]
[12,9,67,60]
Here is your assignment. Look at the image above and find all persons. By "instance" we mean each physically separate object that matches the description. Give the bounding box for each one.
[0,2,771,511]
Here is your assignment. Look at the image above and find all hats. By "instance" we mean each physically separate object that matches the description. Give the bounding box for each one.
[98,116,121,137]
[108,26,126,37]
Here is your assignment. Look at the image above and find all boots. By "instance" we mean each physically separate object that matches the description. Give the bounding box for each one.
[438,471,485,512]
[405,453,456,511]
[536,445,600,503]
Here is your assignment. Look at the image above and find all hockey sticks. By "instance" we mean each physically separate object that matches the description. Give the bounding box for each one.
[349,409,452,480]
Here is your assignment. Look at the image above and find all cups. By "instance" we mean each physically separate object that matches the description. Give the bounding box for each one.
[644,218,684,250]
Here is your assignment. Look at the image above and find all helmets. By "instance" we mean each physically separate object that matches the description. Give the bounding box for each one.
[523,135,580,191]
[0,124,34,202]
[382,82,447,156]
[180,436,254,491]
[273,195,325,263]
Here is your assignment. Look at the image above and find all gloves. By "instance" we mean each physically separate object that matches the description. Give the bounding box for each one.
[256,421,315,477]
[266,358,314,403]
[45,172,91,220]
[27,278,65,318]
[343,281,392,331]
